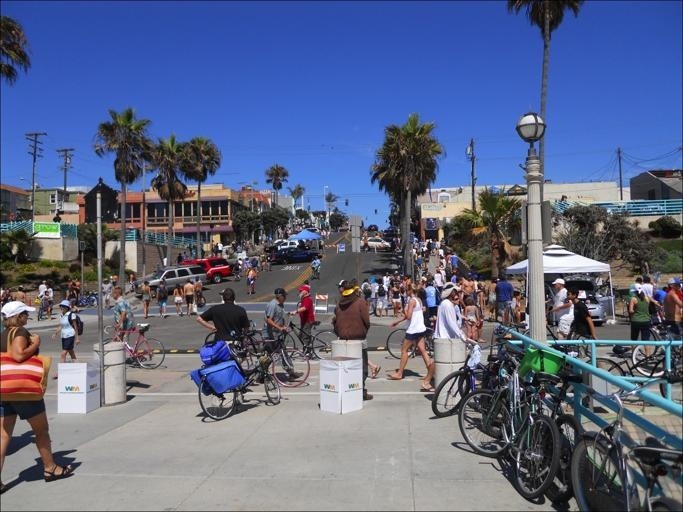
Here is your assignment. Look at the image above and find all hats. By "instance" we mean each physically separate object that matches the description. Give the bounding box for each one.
[219,288,234,295]
[668,278,683,284]
[552,278,565,285]
[441,281,460,298]
[630,284,643,292]
[275,288,288,296]
[335,280,354,288]
[59,300,71,308]
[297,284,311,291]
[1,301,36,317]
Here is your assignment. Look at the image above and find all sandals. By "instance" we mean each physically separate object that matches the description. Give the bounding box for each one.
[44,465,72,482]
[372,365,380,378]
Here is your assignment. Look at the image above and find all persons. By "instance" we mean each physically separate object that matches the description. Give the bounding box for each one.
[0,301,73,495]
[1,208,683,406]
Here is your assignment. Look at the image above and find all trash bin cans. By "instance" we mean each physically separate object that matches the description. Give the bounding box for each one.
[25,296,31,305]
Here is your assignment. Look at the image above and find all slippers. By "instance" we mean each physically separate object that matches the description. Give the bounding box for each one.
[420,387,436,392]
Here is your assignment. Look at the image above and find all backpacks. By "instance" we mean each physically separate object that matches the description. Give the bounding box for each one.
[378,284,385,296]
[158,286,167,300]
[68,312,83,335]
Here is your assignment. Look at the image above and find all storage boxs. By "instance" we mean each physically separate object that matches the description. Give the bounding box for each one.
[317,354,363,413]
[56,362,99,414]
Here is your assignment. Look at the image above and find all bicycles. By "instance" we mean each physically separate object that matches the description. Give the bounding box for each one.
[195,290,206,308]
[103,324,165,369]
[385,319,436,360]
[204,319,265,357]
[278,313,337,360]
[198,340,281,421]
[37,298,45,322]
[71,291,98,308]
[237,329,310,388]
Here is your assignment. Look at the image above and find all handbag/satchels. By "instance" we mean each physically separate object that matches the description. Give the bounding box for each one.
[0,326,52,402]
[191,341,246,395]
[191,304,197,314]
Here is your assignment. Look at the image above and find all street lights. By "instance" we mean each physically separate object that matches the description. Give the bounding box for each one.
[323,184,329,212]
[514,112,550,399]
[465,137,478,212]
[252,178,258,248]
[209,223,214,258]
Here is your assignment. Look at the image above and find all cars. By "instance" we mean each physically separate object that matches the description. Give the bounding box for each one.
[264,246,314,266]
[543,274,616,326]
[277,240,299,250]
[305,227,325,240]
[382,229,393,239]
[359,236,391,252]
[221,246,249,271]
[272,239,287,247]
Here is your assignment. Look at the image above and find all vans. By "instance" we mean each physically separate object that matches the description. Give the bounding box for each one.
[132,263,205,298]
[179,257,232,284]
